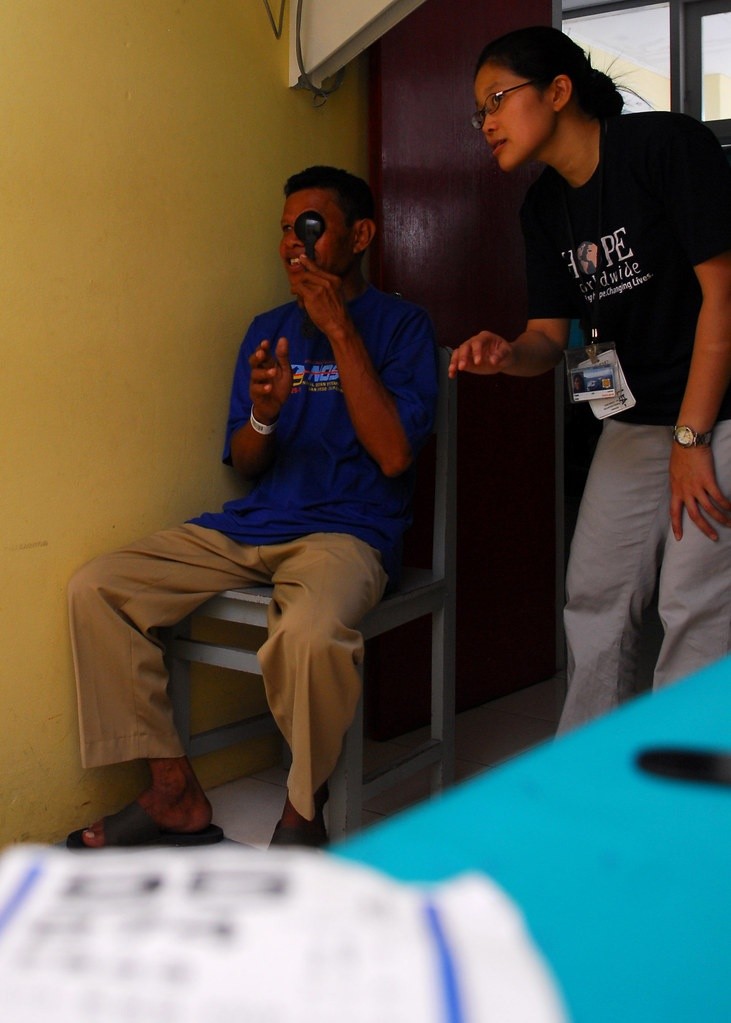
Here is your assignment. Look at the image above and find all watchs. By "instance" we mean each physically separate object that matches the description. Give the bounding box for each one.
[672,423,712,447]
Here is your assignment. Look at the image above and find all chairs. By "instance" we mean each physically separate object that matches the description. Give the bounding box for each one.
[159,344,458,844]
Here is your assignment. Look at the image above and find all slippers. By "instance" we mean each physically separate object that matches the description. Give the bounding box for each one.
[267,783,330,850]
[67,799,224,849]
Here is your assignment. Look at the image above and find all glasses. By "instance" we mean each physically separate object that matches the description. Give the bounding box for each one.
[470,78,535,130]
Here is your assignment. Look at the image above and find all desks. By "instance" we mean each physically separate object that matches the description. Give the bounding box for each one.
[324,657,731,1023]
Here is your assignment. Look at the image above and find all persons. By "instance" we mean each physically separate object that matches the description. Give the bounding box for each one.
[64,163,445,857]
[443,21,730,746]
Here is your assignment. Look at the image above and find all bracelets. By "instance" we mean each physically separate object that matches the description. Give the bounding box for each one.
[248,405,278,437]
